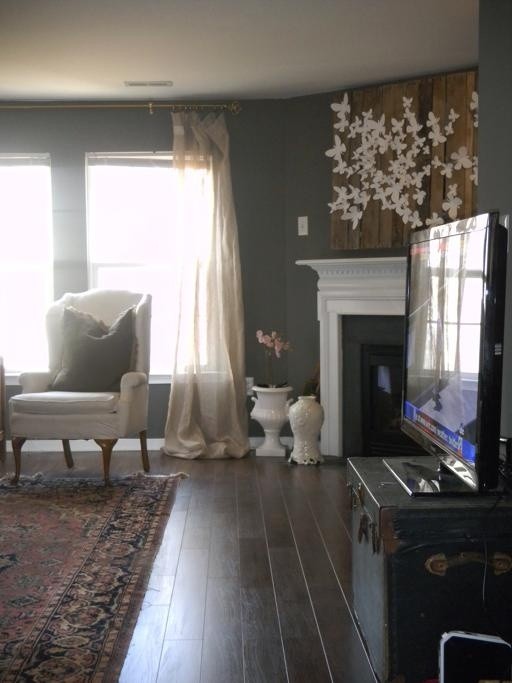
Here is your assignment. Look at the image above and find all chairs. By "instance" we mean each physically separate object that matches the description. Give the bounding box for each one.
[8,289,152,485]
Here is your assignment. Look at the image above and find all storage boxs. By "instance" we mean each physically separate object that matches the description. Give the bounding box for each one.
[347,455,510,683]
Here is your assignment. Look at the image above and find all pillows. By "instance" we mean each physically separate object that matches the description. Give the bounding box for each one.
[51,309,136,393]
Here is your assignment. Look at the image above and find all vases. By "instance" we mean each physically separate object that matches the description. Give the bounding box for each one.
[250,386,293,456]
[289,396,323,465]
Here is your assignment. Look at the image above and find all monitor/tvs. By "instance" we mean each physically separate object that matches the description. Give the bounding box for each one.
[383,210,512,498]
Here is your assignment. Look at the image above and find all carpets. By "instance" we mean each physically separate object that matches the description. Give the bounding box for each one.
[0,474,184,683]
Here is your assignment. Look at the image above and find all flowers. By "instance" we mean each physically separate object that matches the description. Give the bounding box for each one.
[255,330,291,385]
